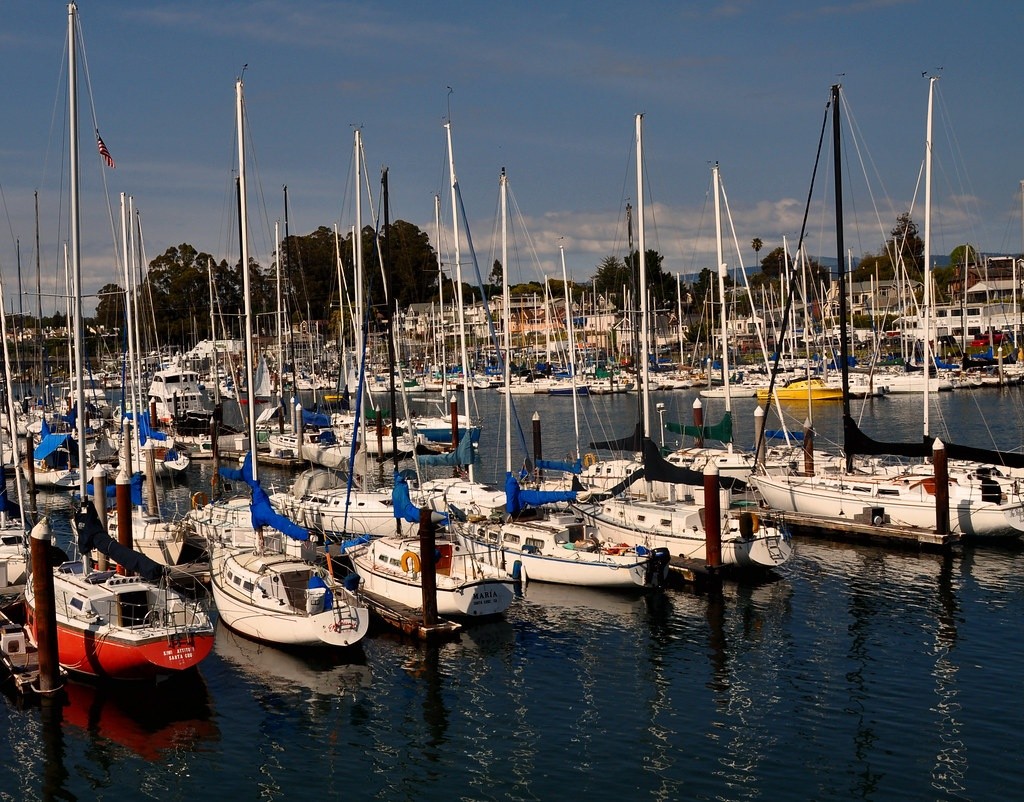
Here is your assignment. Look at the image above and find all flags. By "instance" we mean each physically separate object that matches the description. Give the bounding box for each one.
[97,130,116,170]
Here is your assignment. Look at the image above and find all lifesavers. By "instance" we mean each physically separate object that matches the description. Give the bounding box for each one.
[583,453,596,467]
[401,551,421,573]
[191,492,208,509]
[745,513,760,534]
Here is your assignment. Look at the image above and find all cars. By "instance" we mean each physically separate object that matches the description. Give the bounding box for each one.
[984,330,1008,344]
[533,351,547,360]
[933,336,956,349]
[969,334,991,347]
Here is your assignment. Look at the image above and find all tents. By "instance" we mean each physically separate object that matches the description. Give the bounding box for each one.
[35,433,90,460]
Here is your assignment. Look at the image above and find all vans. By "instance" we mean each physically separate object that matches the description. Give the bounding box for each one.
[741,339,760,351]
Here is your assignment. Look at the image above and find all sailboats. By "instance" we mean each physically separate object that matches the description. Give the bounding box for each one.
[0,1,1024,684]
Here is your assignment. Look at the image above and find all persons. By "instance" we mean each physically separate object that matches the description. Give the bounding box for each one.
[589,533,599,551]
